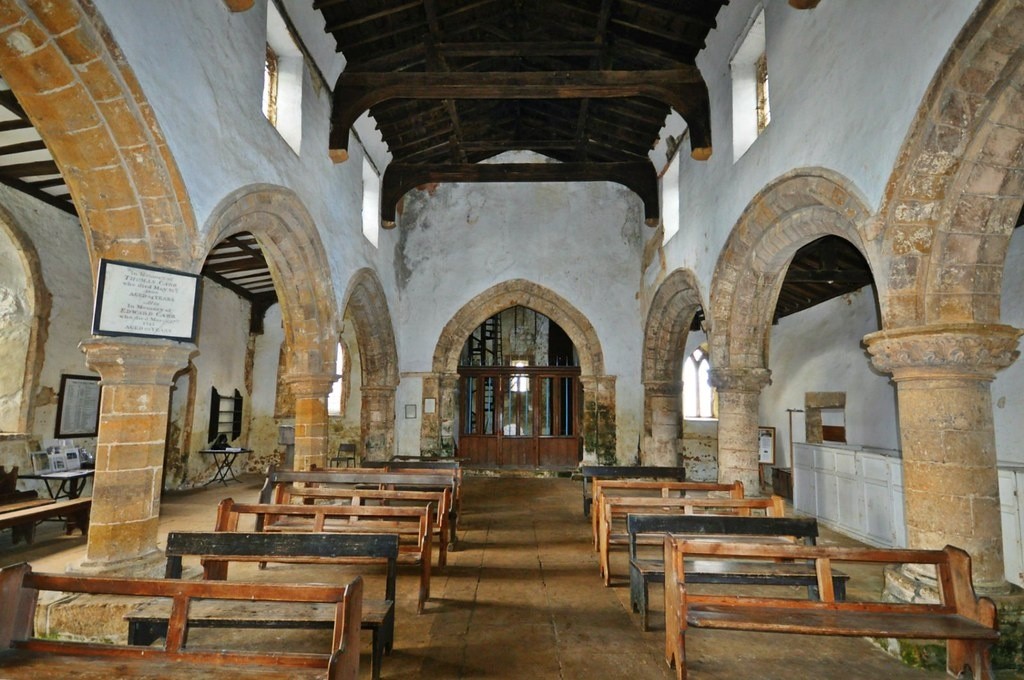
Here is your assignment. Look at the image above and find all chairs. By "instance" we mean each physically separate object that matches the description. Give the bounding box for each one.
[330,443,356,468]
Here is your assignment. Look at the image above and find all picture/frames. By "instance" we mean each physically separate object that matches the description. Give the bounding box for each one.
[54,374,102,439]
[91,257,202,342]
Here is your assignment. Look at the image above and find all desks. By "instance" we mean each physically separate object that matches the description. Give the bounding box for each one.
[199,450,254,487]
[18,468,95,529]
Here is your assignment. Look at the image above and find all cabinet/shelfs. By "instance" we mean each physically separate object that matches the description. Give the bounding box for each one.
[208,386,243,444]
[792,442,1023,589]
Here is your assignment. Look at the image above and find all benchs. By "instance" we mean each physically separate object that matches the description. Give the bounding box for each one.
[0,455,470,680]
[582,466,1001,680]
